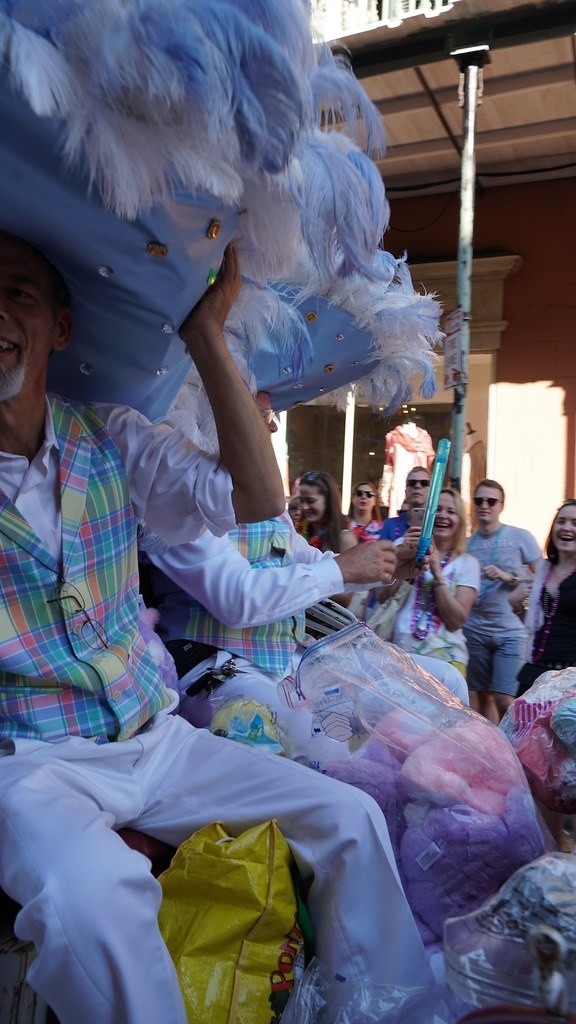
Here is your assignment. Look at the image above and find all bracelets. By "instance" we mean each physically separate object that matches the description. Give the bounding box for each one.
[434,581,446,586]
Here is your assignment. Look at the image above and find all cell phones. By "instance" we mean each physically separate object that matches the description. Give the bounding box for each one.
[410,508,426,537]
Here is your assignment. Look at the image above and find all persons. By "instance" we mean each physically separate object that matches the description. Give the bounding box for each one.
[0,234,433,1024]
[145,388,467,801]
[284,465,576,726]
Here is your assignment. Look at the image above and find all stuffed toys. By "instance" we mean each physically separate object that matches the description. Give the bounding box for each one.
[311,681,545,944]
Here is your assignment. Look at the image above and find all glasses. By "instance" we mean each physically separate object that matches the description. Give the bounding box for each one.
[563,499,576,505]
[472,497,498,506]
[44,579,110,651]
[300,472,320,483]
[261,408,276,424]
[406,479,430,487]
[354,489,376,499]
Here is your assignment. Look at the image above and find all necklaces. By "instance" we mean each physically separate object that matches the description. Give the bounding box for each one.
[542,571,565,618]
[411,549,452,641]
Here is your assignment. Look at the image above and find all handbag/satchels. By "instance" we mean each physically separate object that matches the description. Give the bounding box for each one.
[154,818,302,1024]
